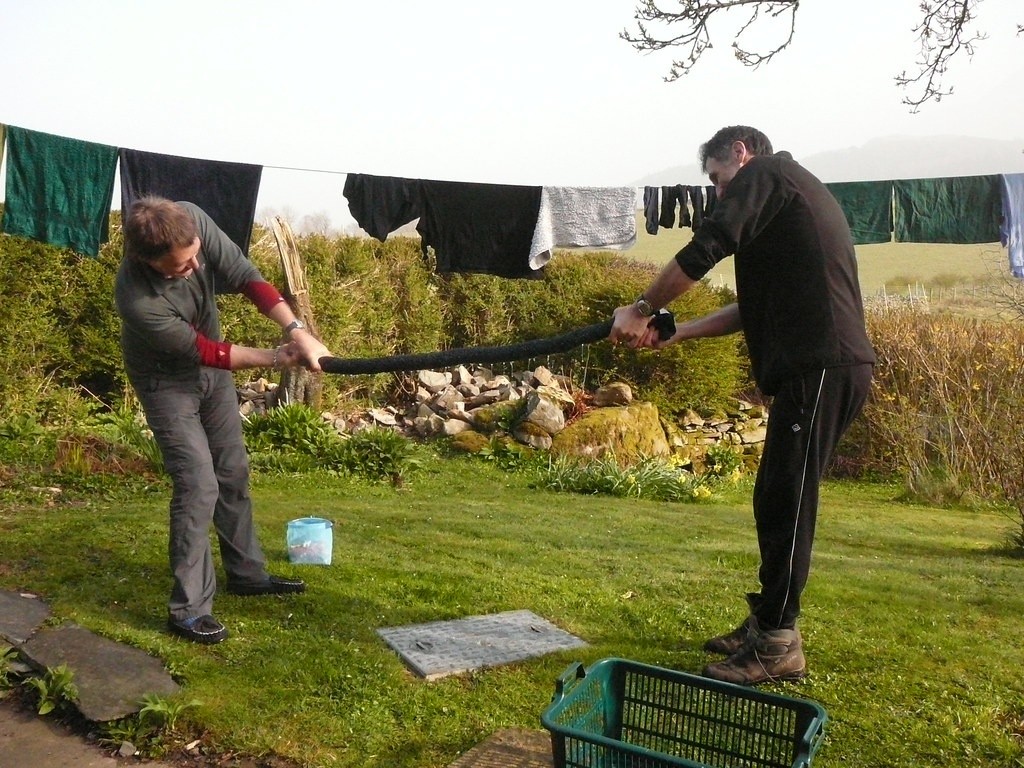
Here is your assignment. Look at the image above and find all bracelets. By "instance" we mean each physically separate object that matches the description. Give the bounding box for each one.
[274,346,280,366]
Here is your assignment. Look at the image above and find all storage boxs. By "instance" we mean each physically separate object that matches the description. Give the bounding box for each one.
[539,656,827,768]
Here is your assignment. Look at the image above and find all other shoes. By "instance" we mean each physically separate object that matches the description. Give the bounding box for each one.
[227,575,306,595]
[168,614,227,643]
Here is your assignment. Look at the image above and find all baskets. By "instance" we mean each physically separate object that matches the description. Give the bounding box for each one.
[542,657,827,768]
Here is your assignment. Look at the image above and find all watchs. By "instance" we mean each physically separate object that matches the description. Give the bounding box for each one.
[636,294,658,316]
[285,319,304,332]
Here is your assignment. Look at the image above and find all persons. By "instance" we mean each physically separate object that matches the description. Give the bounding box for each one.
[611,125,877,684]
[111,195,333,643]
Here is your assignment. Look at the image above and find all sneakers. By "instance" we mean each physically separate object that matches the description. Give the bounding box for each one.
[702,614,807,683]
[707,594,762,654]
